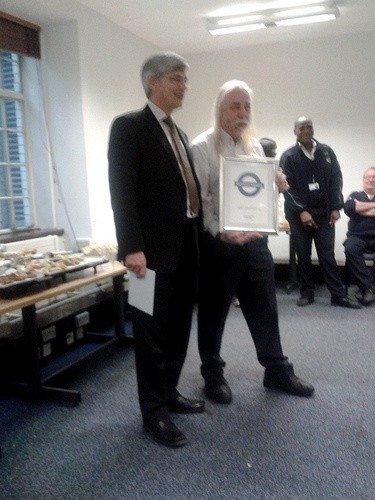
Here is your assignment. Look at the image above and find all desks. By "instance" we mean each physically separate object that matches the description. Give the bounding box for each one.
[0,261,138,406]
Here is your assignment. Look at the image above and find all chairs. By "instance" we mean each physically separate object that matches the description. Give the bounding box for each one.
[345,252,375,296]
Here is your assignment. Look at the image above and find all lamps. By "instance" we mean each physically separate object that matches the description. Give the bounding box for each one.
[208,0,339,35]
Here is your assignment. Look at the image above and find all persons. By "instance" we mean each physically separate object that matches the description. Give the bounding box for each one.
[343,168,375,305]
[189,80,314,404]
[108,53,205,448]
[280,116,355,309]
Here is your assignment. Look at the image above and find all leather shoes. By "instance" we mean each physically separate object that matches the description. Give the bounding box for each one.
[330,295,362,309]
[296,294,315,307]
[264,362,315,396]
[206,375,234,405]
[170,394,208,415]
[363,291,373,304]
[142,413,192,449]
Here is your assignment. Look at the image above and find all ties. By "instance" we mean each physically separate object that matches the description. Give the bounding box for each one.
[164,118,201,217]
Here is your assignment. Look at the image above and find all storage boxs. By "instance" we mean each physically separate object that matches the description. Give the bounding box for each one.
[39,300,99,361]
